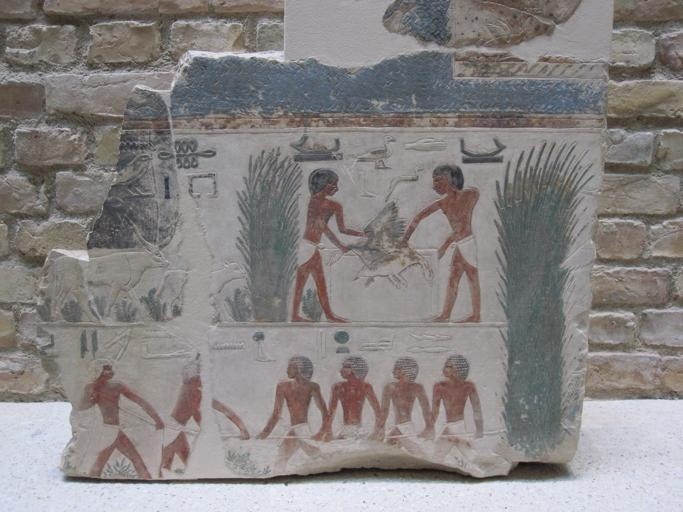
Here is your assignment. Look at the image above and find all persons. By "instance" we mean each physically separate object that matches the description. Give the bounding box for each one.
[365,357,436,455]
[76,359,165,478]
[256,357,334,476]
[291,168,370,323]
[418,355,484,476]
[159,362,248,479]
[394,163,481,325]
[312,356,384,444]
